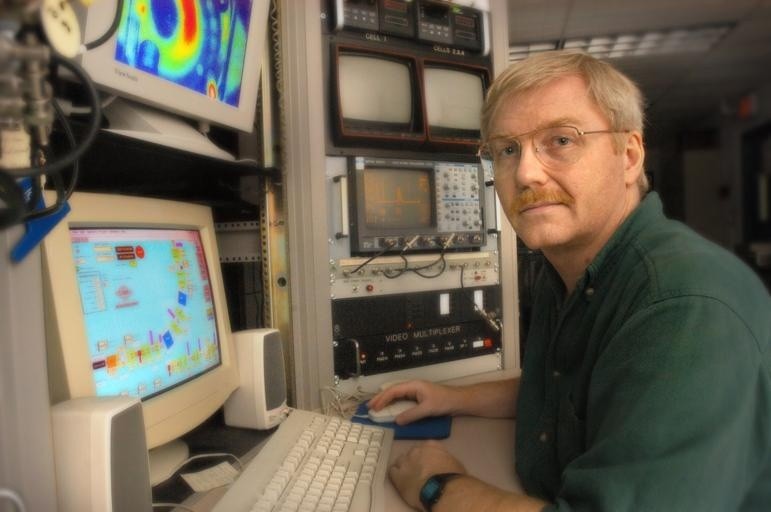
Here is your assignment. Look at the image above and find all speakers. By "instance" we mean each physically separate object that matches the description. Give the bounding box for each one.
[222,328,291,430]
[51,395,153,512]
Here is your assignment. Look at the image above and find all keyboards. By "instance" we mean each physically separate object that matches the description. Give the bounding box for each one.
[208,408,394,512]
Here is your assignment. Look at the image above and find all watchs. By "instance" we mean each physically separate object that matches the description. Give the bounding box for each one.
[419,472,463,511]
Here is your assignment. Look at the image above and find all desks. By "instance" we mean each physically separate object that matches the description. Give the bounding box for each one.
[169,401,527,512]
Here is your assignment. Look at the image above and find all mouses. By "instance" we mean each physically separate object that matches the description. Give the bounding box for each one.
[369,401,417,423]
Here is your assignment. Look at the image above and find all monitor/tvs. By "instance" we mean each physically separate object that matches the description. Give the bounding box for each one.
[42,187,242,490]
[58,0,270,162]
[331,41,425,141]
[421,56,493,148]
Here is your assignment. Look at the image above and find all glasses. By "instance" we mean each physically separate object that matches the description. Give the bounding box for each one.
[477,126,629,179]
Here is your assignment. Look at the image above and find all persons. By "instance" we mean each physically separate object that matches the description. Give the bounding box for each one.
[367,48,771,512]
